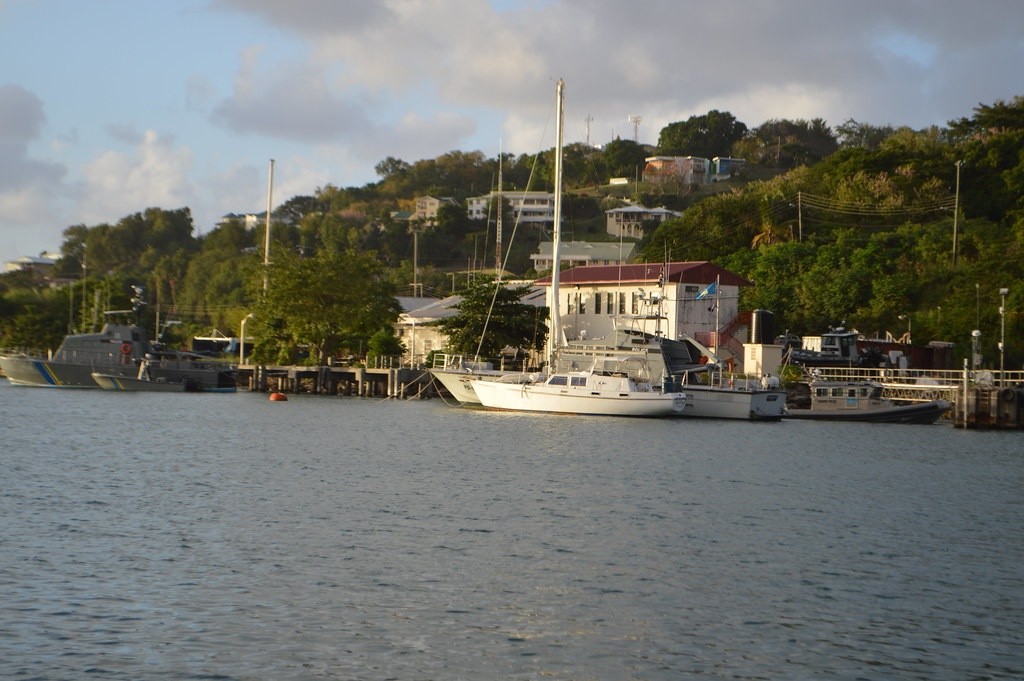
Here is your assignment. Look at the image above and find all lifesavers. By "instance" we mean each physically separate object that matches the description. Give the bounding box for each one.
[120,343,132,355]
[724,359,732,372]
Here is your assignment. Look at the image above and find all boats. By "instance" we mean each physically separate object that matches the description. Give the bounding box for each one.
[470,372,687,417]
[790,332,881,368]
[783,381,952,424]
[91,360,186,392]
[555,314,787,422]
[0,310,237,393]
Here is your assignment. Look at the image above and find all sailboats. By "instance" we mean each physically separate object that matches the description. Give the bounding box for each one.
[431,83,643,409]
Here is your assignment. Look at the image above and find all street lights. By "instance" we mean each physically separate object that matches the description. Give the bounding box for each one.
[1000,288,1009,388]
[239,314,255,365]
[898,315,911,331]
[398,313,415,370]
